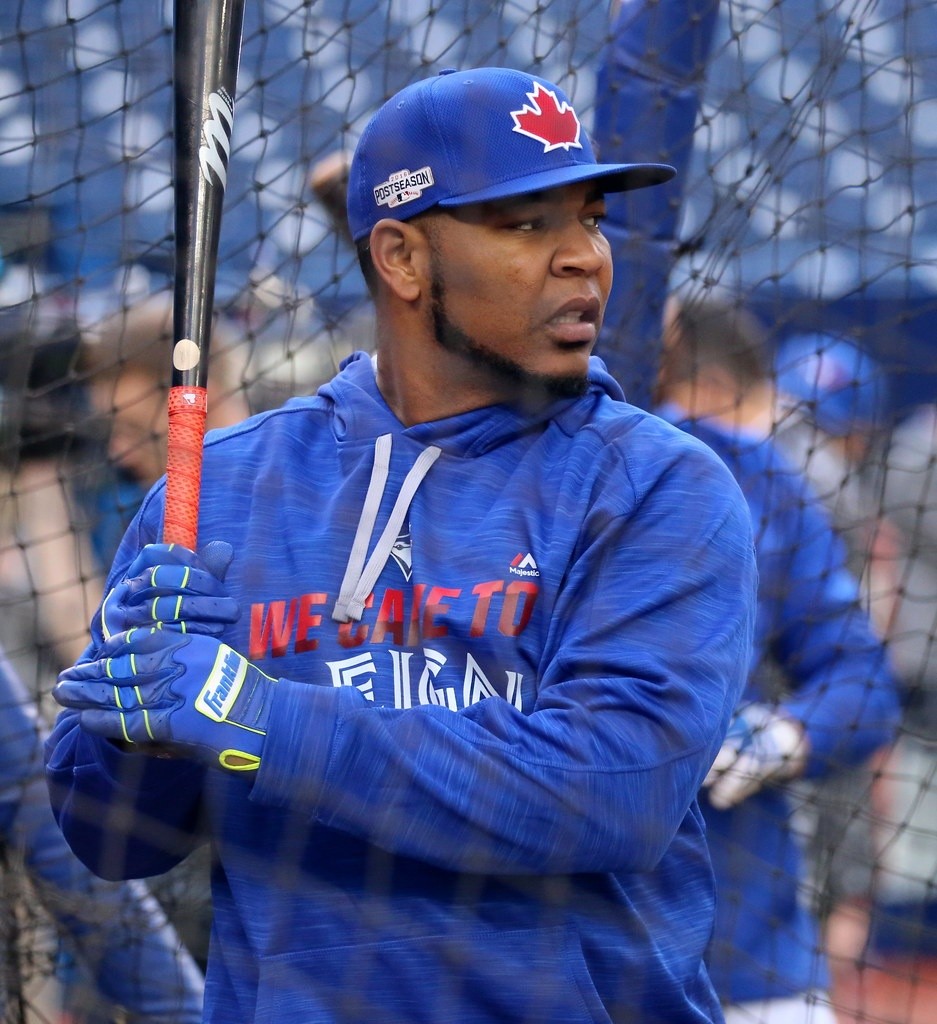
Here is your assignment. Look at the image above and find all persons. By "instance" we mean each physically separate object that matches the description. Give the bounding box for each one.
[0,291,243,1024]
[648,396,898,1024]
[657,246,898,969]
[40,64,761,1024]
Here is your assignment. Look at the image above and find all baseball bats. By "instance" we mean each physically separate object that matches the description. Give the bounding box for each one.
[137,1,248,763]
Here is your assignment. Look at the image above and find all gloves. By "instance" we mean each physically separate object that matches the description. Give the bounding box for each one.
[55,541,279,778]
[701,701,810,810]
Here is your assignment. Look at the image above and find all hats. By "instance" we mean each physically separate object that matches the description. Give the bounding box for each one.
[345,68,676,242]
[776,337,891,435]
